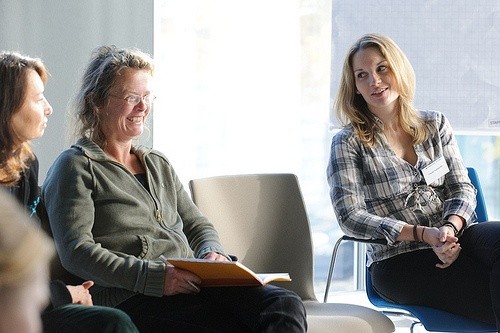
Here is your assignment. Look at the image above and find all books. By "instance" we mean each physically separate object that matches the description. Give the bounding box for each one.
[160,255,291,288]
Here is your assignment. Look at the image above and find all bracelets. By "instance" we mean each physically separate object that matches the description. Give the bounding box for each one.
[421,226,426,243]
[444,221,459,236]
[412,225,420,243]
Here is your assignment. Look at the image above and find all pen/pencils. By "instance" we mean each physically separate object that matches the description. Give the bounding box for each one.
[159,254,199,291]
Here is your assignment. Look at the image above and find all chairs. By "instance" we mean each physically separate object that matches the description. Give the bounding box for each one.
[189,173,396,333]
[323,166,500,333]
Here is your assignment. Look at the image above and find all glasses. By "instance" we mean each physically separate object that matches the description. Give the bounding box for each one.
[108,93,158,105]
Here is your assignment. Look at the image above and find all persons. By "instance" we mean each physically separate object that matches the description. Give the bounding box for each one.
[327,32,500,330]
[42,46,308,333]
[0,52,137,333]
